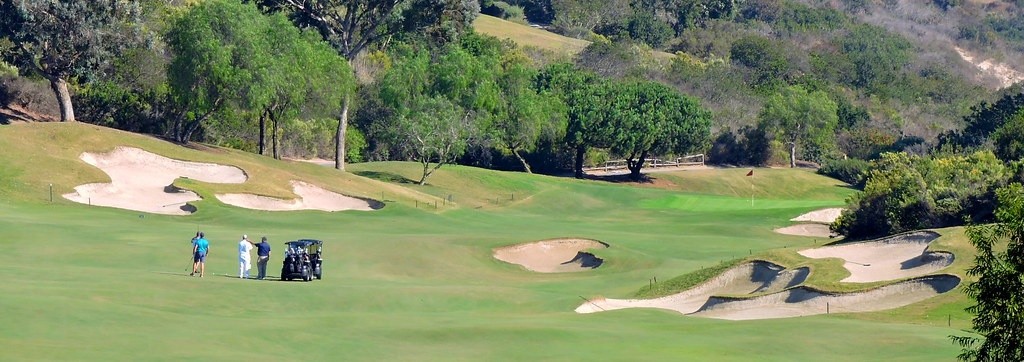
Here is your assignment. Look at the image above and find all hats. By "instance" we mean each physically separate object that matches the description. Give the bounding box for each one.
[243,234,248,239]
[261,236,267,241]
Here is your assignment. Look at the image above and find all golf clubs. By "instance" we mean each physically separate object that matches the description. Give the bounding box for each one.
[184,254,195,271]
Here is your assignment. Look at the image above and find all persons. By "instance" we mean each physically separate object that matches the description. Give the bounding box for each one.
[248,236,271,279]
[286,248,311,260]
[238,234,253,279]
[190,231,210,277]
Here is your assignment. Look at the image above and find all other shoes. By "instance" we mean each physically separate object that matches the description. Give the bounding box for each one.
[193,273,195,276]
[195,270,200,273]
[190,272,193,275]
[200,274,203,278]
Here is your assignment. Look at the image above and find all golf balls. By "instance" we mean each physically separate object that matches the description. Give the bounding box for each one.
[213,273,214,275]
[226,273,227,275]
